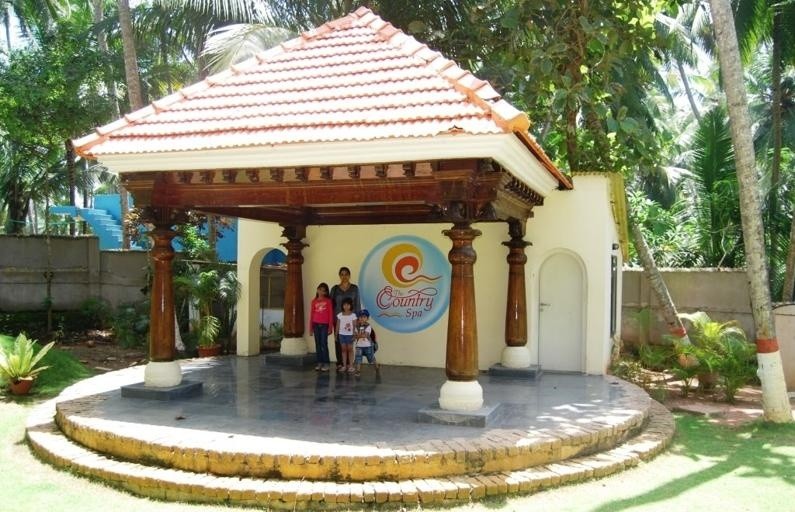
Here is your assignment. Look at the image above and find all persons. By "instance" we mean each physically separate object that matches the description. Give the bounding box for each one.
[348,309,382,378]
[334,298,358,373]
[309,282,334,372]
[329,267,362,370]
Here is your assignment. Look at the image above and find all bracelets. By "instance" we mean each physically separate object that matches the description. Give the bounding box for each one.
[335,335,337,336]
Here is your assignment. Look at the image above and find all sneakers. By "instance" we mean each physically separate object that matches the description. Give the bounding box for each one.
[315,363,355,372]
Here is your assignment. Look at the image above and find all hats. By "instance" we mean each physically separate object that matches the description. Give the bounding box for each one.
[359,310,369,316]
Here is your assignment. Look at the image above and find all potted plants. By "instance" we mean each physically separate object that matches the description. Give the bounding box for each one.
[674,311,749,390]
[195,315,222,358]
[0,330,57,397]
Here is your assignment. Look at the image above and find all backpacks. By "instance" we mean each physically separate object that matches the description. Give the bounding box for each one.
[356,323,378,353]
